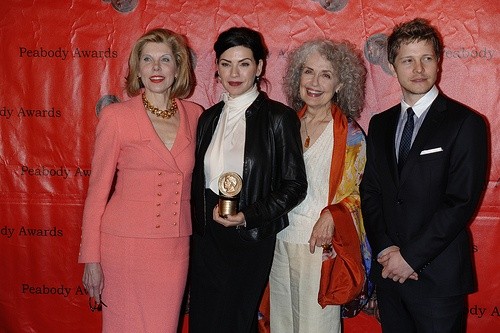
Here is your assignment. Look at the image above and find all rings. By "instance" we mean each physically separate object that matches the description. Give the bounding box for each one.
[321,242,331,248]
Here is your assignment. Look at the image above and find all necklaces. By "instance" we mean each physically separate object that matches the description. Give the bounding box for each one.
[304,112,330,148]
[141,92,178,119]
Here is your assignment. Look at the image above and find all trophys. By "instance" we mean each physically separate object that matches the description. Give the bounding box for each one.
[217,172,242,218]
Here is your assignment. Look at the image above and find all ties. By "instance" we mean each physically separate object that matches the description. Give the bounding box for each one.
[397,107,415,172]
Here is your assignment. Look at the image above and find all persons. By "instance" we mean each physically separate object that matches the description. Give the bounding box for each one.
[78,28,203,333]
[359,17,492,333]
[189,27,308,333]
[267,39,367,333]
